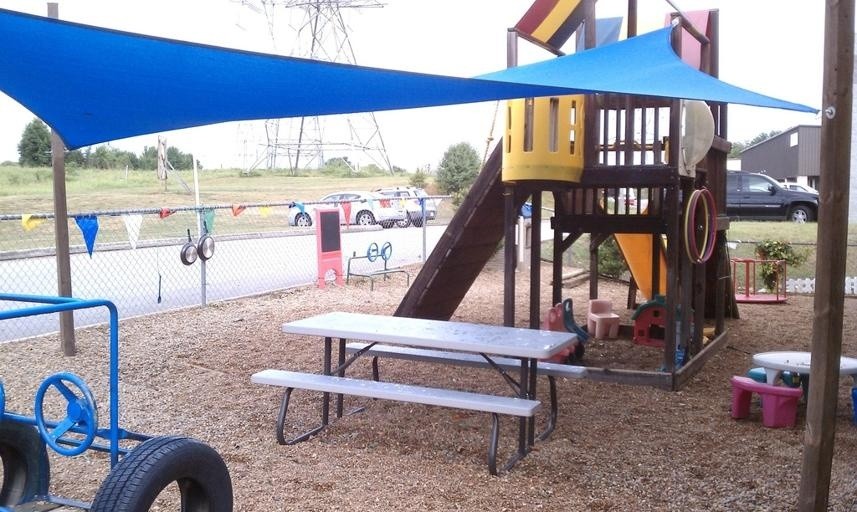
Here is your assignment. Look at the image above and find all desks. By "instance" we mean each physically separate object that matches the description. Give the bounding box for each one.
[751,349,857,386]
[282,308,579,450]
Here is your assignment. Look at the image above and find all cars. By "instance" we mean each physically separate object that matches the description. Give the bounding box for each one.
[727,170,819,225]
[289,186,436,228]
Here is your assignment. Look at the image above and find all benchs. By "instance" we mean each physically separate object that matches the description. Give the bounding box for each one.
[249,368,541,475]
[345,254,410,292]
[344,342,588,425]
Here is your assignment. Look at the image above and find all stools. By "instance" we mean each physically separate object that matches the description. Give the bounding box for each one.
[587,299,620,340]
[545,298,587,359]
[727,375,800,428]
